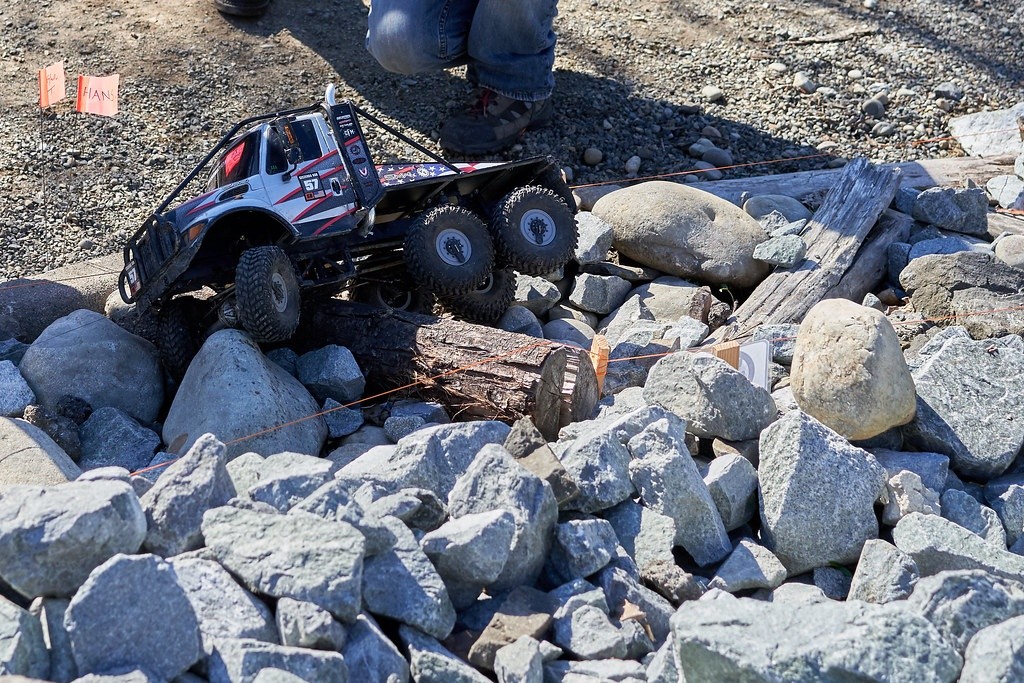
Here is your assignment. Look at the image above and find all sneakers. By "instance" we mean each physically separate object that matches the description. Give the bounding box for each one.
[440,90,552,154]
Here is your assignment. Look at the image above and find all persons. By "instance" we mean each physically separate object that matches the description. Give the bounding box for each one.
[210,0,559,157]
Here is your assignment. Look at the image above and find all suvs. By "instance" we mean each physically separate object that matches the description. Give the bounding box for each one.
[118,83,583,380]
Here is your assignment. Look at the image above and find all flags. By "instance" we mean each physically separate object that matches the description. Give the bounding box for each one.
[39,63,66,109]
[76,74,120,118]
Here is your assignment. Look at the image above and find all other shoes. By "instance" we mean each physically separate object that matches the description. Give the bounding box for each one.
[214,0,269,16]
